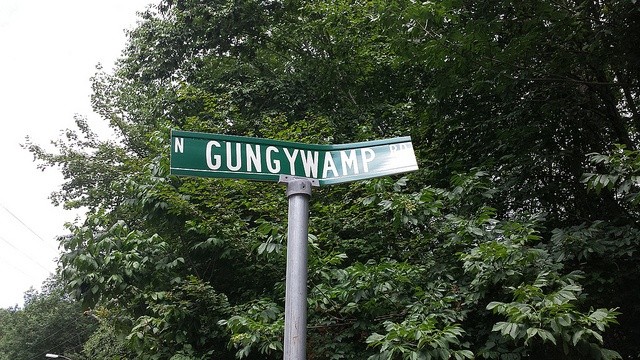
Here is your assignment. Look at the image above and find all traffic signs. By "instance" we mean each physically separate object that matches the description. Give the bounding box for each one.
[161,128,422,191]
